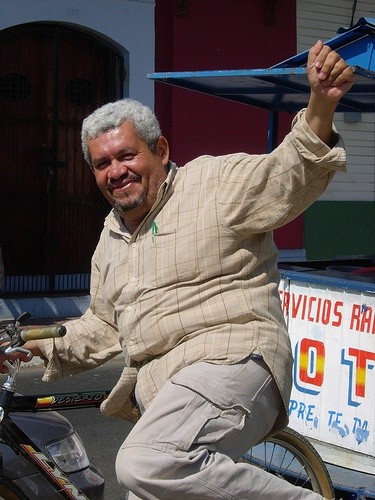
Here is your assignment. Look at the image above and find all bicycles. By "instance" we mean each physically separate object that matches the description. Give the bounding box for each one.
[0,310,336,500]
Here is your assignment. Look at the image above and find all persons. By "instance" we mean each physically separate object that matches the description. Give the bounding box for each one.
[1,39,355,500]
